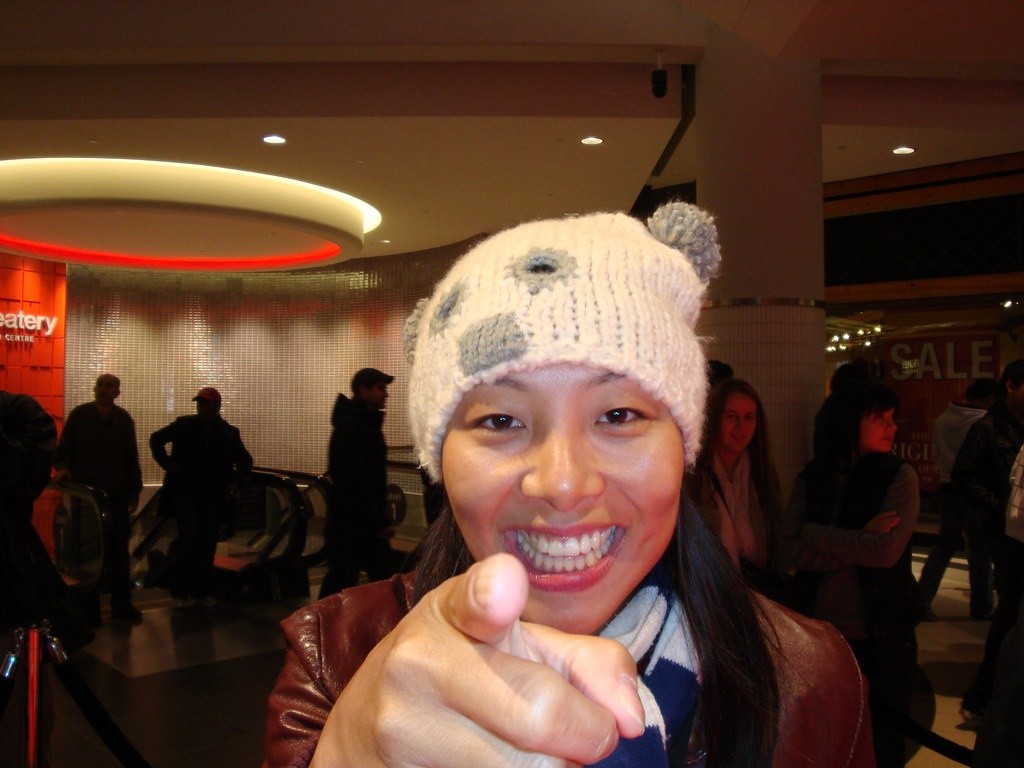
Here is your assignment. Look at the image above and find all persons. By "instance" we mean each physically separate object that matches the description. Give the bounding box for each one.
[0,390,58,623]
[149,387,253,610]
[265,199,876,768]
[318,368,402,600]
[54,373,144,628]
[686,361,1024,768]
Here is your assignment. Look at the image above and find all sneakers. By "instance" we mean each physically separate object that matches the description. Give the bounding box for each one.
[957,705,981,730]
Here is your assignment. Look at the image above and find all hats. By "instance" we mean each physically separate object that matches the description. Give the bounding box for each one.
[400,201,721,483]
[192,387,222,404]
[351,369,394,392]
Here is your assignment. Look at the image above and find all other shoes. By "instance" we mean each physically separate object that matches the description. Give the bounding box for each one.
[110,606,143,621]
[969,606,997,622]
[916,606,940,623]
